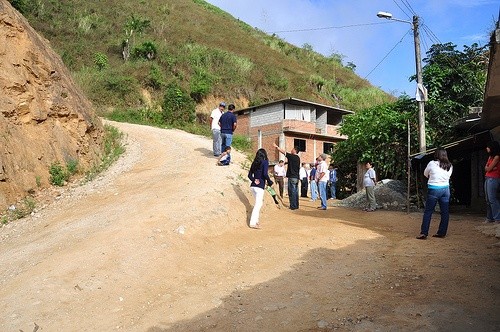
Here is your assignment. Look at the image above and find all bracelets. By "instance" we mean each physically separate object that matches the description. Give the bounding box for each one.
[276,146,278,149]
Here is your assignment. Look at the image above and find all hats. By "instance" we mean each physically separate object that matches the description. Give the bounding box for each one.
[321,153,326,159]
[220,102,225,106]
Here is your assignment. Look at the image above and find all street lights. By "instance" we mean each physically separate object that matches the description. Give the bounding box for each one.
[377,11,427,153]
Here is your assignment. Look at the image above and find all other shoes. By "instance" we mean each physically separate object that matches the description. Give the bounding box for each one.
[367,208,375,212]
[362,208,369,211]
[416,234,425,238]
[251,224,261,229]
[318,207,326,210]
[432,234,444,238]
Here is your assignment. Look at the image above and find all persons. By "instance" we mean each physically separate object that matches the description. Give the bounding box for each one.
[416,147,454,240]
[328,163,337,200]
[362,160,377,212]
[218,104,237,156]
[265,141,300,210]
[316,154,330,210]
[299,162,309,198]
[248,148,275,229]
[209,102,226,157]
[482,143,500,225]
[215,146,231,166]
[309,162,318,201]
